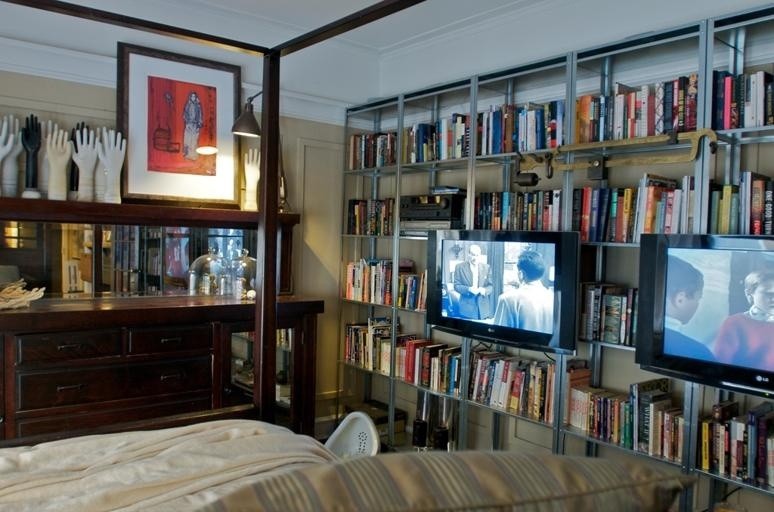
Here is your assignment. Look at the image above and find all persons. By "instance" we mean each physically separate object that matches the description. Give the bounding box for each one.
[713,269,774,372]
[490,251,552,334]
[0,112,127,206]
[664,255,716,360]
[242,148,261,212]
[453,246,494,321]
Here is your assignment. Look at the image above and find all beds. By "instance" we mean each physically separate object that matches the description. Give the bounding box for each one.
[0,417,336,511]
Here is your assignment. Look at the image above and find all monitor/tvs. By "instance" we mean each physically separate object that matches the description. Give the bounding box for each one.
[426,229,580,356]
[635,233,774,402]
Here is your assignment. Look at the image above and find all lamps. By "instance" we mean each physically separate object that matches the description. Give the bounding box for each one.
[230,87,293,216]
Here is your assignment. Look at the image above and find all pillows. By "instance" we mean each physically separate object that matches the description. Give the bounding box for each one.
[188,450,697,510]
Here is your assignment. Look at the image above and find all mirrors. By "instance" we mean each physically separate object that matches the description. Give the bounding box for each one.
[227,328,297,434]
[0,217,278,297]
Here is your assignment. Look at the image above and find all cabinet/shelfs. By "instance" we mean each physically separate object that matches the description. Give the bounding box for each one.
[1,196,326,439]
[331,5,773,510]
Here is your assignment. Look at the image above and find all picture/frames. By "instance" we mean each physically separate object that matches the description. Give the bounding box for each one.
[114,40,243,211]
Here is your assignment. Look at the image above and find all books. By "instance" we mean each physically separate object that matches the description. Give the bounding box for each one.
[343,316,555,424]
[346,172,774,244]
[561,358,774,491]
[337,67,774,172]
[345,259,429,312]
[577,281,638,347]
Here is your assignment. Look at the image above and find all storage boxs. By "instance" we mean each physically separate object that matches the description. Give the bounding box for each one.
[342,398,410,439]
